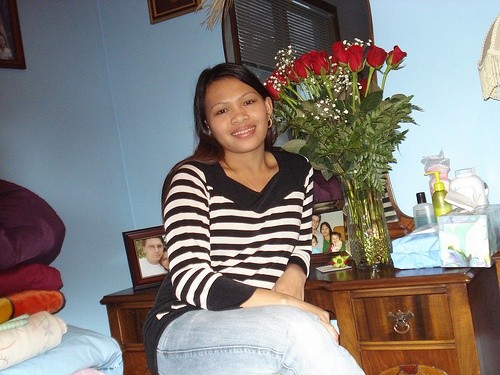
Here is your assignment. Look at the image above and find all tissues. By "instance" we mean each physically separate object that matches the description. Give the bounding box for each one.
[437,175,500,268]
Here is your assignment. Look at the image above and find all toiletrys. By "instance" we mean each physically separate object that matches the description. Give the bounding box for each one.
[423,171,453,223]
[413,192,437,229]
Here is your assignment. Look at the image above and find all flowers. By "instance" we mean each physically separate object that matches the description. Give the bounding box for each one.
[262,36,424,193]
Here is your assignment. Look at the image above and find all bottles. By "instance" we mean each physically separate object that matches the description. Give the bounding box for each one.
[413,192,436,228]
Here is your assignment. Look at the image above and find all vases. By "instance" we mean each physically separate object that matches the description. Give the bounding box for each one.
[341,187,393,270]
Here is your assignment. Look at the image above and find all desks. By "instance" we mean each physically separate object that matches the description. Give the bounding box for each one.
[98,262,478,375]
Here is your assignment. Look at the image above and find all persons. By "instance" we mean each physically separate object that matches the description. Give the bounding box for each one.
[139,236,168,278]
[143,63,367,375]
[312,214,347,254]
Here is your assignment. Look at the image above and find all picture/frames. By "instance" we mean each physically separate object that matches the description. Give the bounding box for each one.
[0,0,28,70]
[311,200,346,263]
[146,0,203,26]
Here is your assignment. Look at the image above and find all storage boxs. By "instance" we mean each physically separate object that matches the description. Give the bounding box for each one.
[121,226,170,292]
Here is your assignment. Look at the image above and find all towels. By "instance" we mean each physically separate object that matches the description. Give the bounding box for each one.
[0,311,68,371]
[0,314,29,330]
